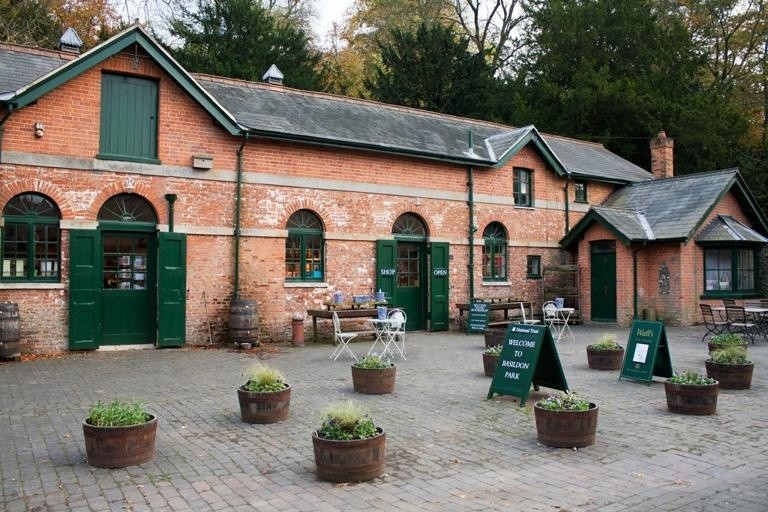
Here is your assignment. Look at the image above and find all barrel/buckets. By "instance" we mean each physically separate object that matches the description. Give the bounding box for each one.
[229,299,259,342]
[0,302,21,356]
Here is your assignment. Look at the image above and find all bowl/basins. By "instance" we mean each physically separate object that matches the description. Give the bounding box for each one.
[351,295,370,303]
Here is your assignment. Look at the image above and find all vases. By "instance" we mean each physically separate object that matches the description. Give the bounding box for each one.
[556,298,564,309]
[333,290,388,320]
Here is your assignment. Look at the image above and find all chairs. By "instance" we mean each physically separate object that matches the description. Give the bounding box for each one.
[520,301,576,344]
[330,309,408,363]
[699,299,768,344]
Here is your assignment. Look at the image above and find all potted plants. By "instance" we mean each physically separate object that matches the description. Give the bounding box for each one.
[705,346,754,389]
[237,361,291,423]
[311,402,386,483]
[707,332,747,355]
[351,352,397,395]
[533,391,600,449]
[664,367,720,414]
[82,399,157,469]
[586,332,625,370]
[483,343,504,377]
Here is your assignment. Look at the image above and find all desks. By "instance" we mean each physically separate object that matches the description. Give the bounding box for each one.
[456,299,535,330]
[307,308,404,346]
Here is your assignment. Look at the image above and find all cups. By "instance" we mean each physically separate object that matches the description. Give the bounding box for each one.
[333,293,341,304]
[376,306,387,321]
[555,297,564,309]
[375,292,384,301]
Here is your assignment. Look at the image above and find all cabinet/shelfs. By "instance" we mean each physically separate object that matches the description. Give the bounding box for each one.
[541,263,581,324]
